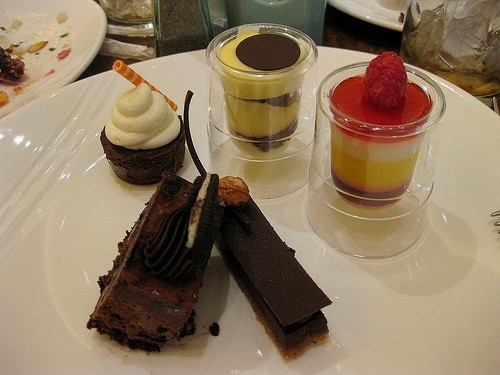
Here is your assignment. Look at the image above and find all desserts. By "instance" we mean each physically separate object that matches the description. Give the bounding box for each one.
[86,90,333,363]
[330,52,431,209]
[100,60,186,186]
[218,33,308,158]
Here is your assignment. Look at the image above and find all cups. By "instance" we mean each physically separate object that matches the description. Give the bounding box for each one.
[306,61,447,257]
[98,0,156,61]
[399,0,500,115]
[205,23,319,200]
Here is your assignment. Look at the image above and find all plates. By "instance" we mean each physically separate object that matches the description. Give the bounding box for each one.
[0,46,500,375]
[327,0,406,32]
[0,0,108,118]
